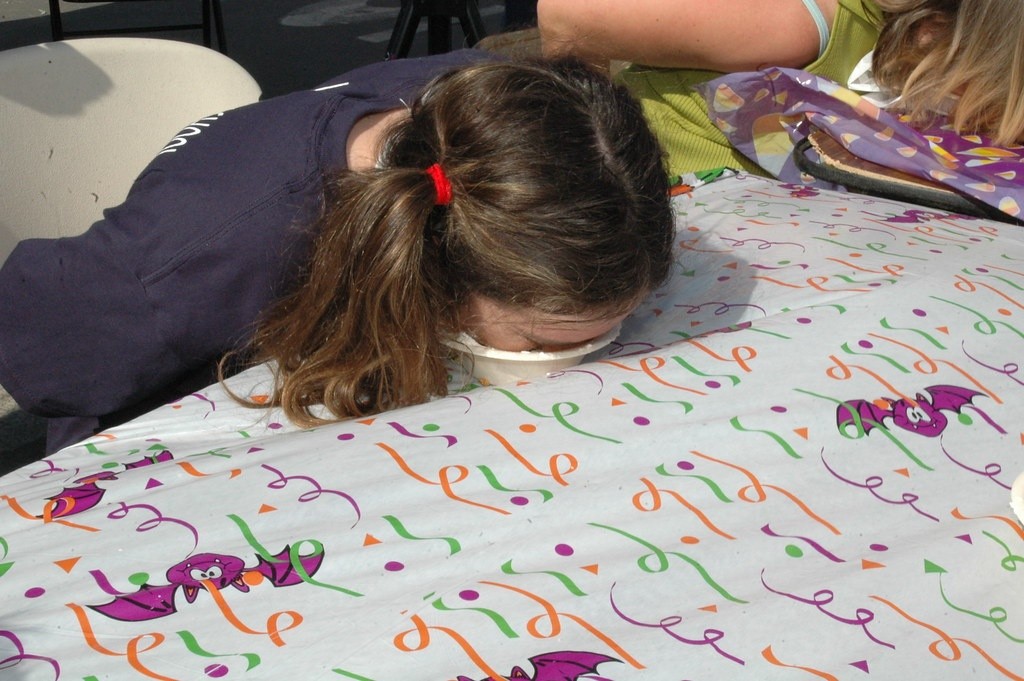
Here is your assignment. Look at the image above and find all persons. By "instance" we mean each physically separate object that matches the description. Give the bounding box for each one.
[0,46,674,458]
[537,0,1024,179]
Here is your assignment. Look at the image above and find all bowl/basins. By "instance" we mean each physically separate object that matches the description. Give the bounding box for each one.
[436,323,622,385]
[918,81,962,119]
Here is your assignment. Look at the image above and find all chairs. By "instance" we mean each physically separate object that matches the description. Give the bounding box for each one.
[0,37,262,453]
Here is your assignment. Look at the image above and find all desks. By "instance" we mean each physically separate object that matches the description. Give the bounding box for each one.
[0,64,1024,681]
